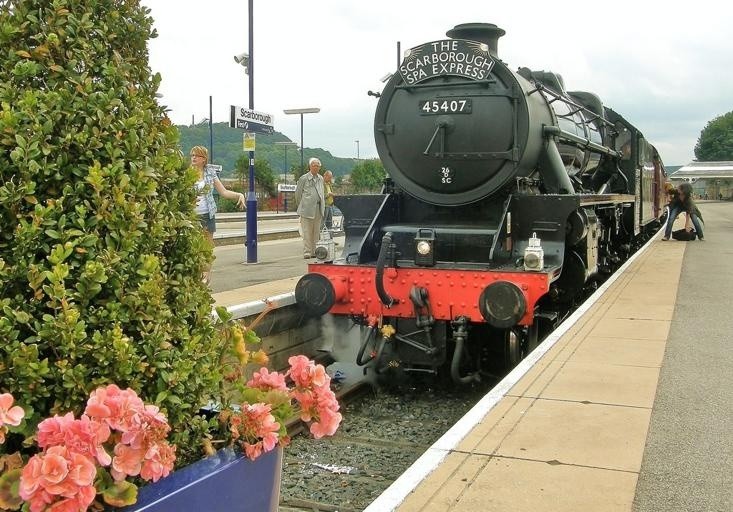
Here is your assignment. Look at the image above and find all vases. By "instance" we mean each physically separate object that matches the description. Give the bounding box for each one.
[116,406,283,512]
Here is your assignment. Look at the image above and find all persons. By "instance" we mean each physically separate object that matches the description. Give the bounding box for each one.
[319,170,335,240]
[620,143,632,159]
[691,192,722,200]
[294,157,325,259]
[183,145,247,287]
[661,182,705,243]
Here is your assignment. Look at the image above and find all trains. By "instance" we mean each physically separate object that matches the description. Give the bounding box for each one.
[293,23,673,396]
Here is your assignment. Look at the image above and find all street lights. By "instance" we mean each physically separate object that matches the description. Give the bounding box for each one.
[282,107,320,174]
[148,93,163,101]
[354,139,361,167]
[273,140,296,213]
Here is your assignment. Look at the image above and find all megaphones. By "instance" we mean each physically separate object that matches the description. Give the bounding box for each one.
[234,51,247,63]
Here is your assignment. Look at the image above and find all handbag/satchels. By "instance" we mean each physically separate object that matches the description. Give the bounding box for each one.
[672,227,697,241]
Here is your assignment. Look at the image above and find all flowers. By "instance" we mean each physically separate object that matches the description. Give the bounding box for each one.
[0,354,345,512]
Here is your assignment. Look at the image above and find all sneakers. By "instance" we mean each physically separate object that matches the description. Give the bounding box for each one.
[662,236,668,241]
[700,238,706,241]
[304,255,316,259]
